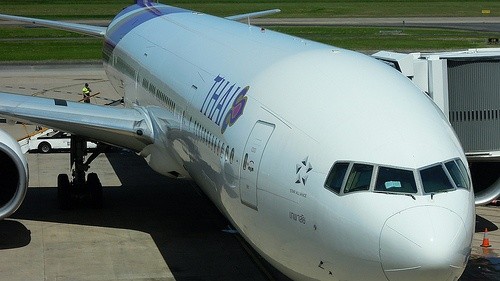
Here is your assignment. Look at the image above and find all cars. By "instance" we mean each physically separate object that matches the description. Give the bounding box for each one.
[28,130,99,154]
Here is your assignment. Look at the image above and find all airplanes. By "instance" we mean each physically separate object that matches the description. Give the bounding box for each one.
[0,1,500,280]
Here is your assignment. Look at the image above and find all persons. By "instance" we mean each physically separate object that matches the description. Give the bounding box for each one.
[83,82,91,103]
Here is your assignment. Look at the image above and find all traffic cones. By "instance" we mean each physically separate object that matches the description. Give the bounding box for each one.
[480,227,492,248]
